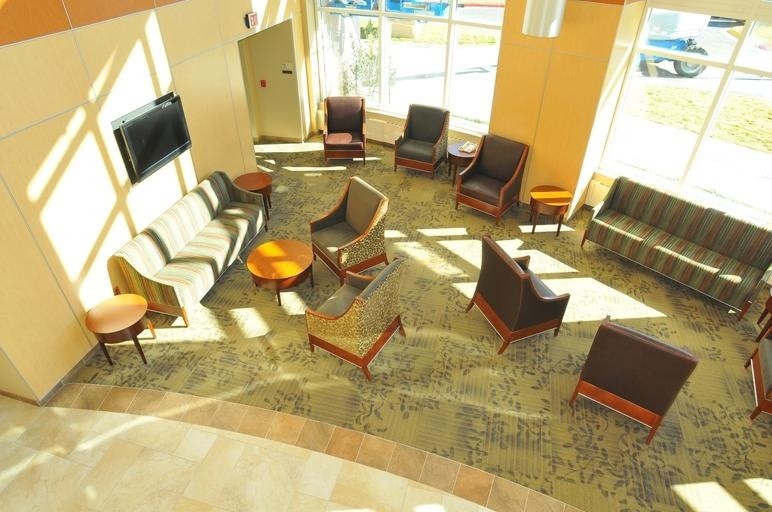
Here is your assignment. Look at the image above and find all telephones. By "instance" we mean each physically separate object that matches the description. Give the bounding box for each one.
[458,141,477,153]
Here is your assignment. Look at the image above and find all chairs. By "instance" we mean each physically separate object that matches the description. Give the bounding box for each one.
[455,133,531,226]
[568,315,699,445]
[465,234,571,356]
[304,255,408,384]
[321,94,367,165]
[393,102,450,179]
[308,173,390,287]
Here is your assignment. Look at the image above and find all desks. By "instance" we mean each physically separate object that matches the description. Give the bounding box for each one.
[244,238,317,308]
[231,170,274,220]
[84,293,158,366]
[447,141,476,188]
[755,287,772,341]
[528,184,572,236]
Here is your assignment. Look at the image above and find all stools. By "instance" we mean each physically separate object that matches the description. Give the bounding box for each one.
[744,326,772,420]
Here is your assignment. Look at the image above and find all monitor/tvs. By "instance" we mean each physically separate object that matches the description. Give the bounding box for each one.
[118,95,193,183]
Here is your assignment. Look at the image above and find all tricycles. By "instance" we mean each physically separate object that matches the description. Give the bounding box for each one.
[637,8,711,75]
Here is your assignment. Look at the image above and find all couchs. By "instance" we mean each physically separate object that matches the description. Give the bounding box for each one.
[581,175,772,321]
[112,169,269,329]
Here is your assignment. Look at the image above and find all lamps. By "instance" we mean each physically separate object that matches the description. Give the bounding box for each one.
[521,0,566,38]
